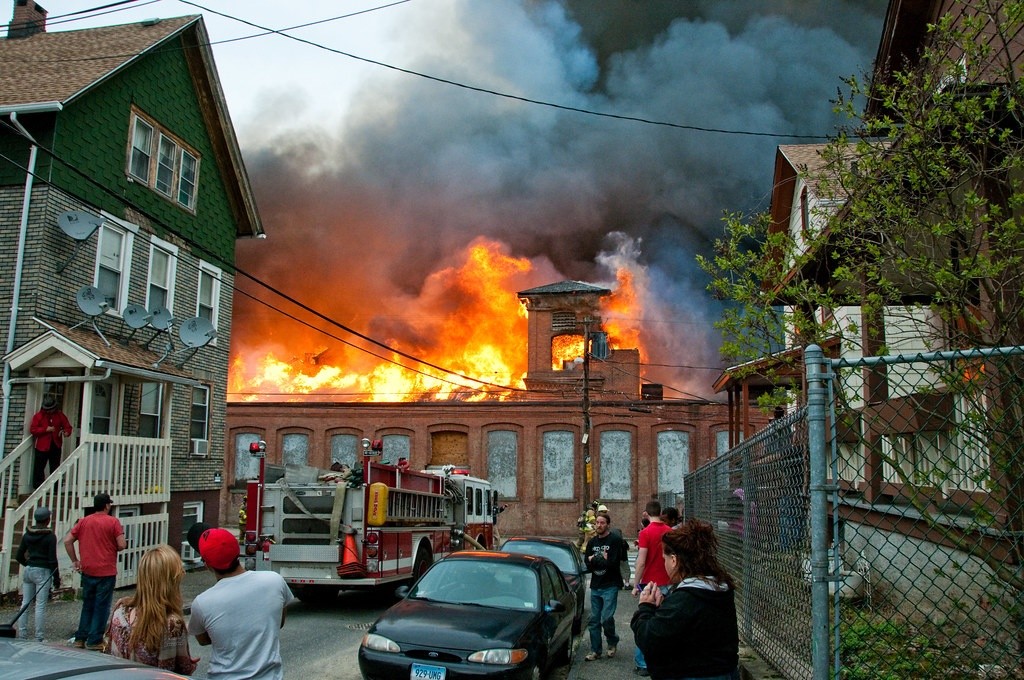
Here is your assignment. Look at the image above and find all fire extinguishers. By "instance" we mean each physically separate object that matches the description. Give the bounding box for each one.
[261,538,276,561]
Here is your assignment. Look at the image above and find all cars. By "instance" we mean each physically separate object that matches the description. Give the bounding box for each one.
[796,546,865,599]
[718,482,802,541]
[0,635,193,680]
[492,534,586,633]
[359,550,579,679]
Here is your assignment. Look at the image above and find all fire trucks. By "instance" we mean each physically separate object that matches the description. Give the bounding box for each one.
[245,438,510,610]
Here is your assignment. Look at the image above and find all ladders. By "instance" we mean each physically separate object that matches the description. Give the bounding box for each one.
[385,487,451,524]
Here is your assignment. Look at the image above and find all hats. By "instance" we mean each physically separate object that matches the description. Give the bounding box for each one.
[41,396,56,409]
[597,504,610,512]
[94,493,113,506]
[186,522,239,569]
[34,507,53,521]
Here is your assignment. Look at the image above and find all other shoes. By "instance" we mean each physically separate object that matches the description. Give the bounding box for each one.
[585,651,601,661]
[606,646,616,657]
[74,639,108,650]
[625,584,634,590]
[633,666,650,676]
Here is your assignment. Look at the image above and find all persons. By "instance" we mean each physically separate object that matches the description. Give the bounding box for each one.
[236,496,247,545]
[492,503,509,550]
[576,499,684,677]
[103,544,201,676]
[187,528,300,680]
[63,494,126,651]
[29,396,72,495]
[16,507,61,643]
[630,520,740,680]
